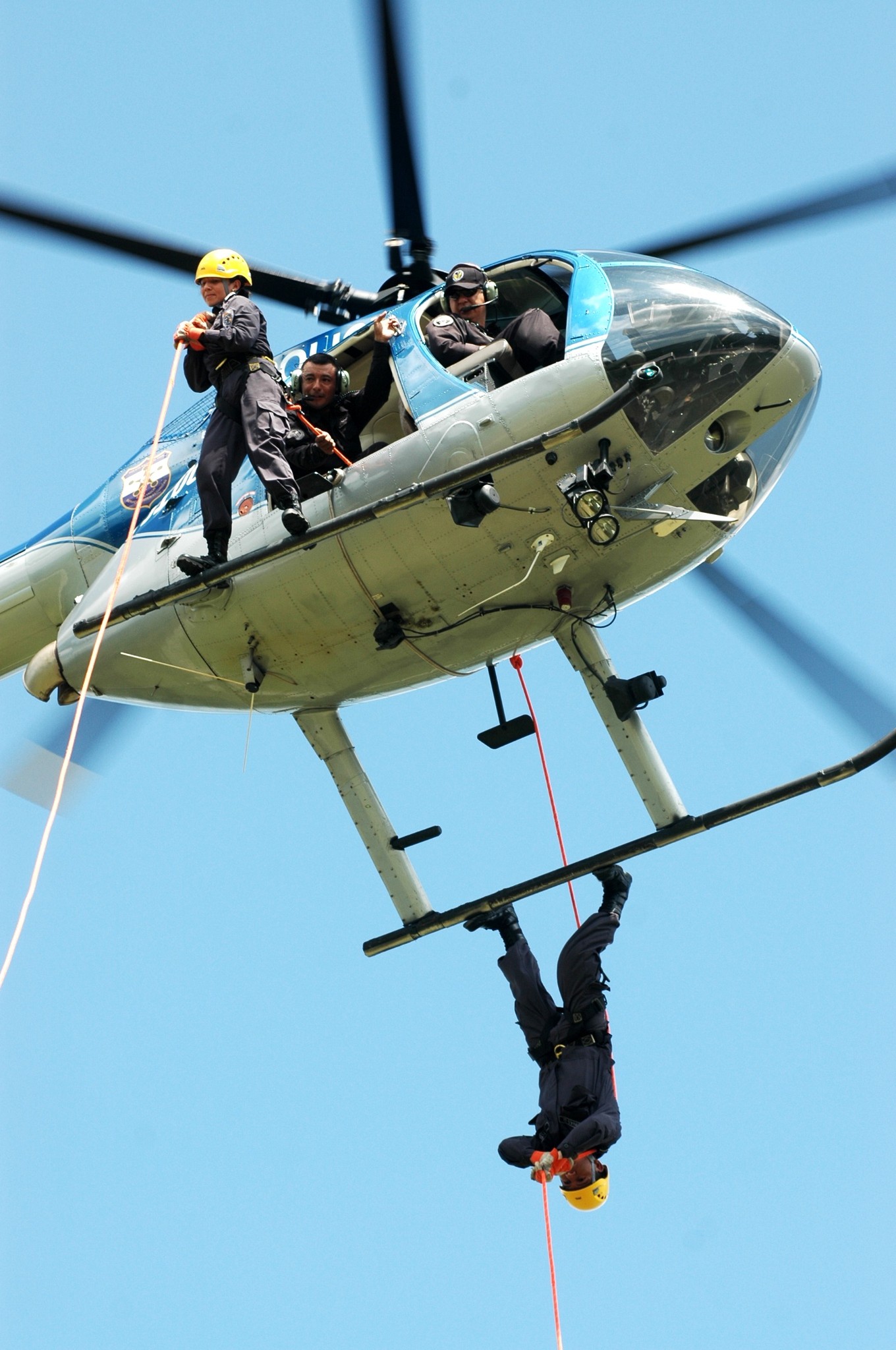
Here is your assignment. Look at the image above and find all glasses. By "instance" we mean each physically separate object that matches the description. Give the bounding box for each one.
[447,285,484,299]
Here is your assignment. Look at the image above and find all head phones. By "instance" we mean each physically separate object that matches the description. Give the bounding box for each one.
[440,263,499,313]
[290,353,349,393]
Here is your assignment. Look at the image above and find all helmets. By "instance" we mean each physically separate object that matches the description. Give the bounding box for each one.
[195,248,253,287]
[559,1165,610,1211]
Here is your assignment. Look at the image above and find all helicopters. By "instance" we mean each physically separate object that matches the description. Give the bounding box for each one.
[1,0,893,959]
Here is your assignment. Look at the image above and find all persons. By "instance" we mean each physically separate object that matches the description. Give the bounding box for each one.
[462,864,633,1212]
[282,310,402,489]
[422,261,565,388]
[172,248,317,590]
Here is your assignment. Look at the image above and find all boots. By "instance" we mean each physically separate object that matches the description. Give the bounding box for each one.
[463,904,526,953]
[177,537,229,588]
[592,865,632,920]
[275,485,317,550]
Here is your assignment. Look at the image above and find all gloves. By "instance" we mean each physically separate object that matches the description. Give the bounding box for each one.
[173,322,205,350]
[190,310,214,331]
[530,1149,574,1183]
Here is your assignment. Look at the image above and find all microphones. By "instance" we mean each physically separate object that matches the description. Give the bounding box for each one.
[462,296,498,312]
[291,391,314,401]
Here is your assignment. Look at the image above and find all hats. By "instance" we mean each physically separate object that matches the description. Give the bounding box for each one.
[445,263,488,297]
